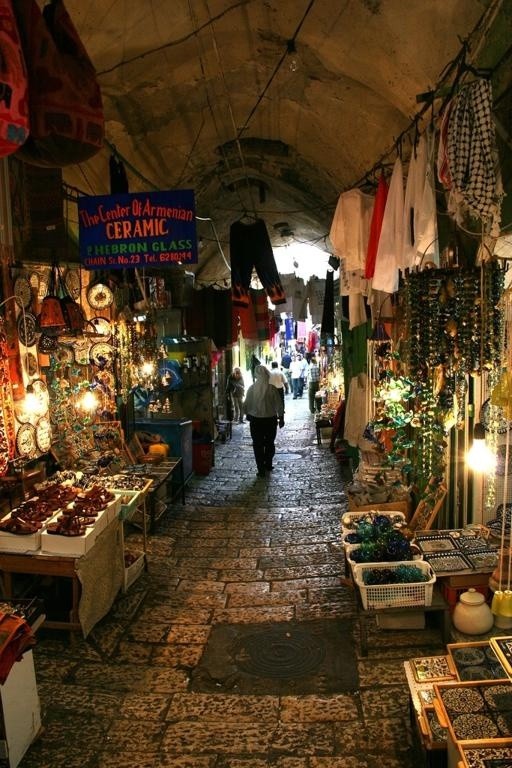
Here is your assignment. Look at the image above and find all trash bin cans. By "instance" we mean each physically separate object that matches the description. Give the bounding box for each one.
[192,441,213,475]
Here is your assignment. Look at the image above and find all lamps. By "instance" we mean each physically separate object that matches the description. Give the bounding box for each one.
[273,222,294,242]
[285,39,302,73]
[0,295,40,413]
[465,424,496,473]
[367,294,396,340]
[81,328,97,413]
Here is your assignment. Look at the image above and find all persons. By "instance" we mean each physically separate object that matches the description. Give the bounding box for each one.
[242,365,285,479]
[305,357,323,414]
[279,339,330,399]
[224,368,246,424]
[269,362,289,421]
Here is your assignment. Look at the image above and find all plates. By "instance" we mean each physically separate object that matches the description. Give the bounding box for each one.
[479,396,512,434]
[15,266,115,458]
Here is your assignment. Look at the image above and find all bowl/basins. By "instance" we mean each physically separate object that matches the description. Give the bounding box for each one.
[137,454,164,465]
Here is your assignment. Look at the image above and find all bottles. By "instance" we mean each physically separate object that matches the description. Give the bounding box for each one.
[180,355,208,387]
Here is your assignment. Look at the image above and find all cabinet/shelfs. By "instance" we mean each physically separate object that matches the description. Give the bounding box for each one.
[162,336,215,477]
[134,418,196,487]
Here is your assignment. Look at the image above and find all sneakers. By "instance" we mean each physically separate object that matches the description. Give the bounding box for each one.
[256,466,275,477]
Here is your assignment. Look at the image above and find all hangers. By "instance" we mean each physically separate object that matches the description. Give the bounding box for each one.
[234,208,259,224]
[357,44,491,194]
[327,265,335,272]
[209,277,229,291]
[356,365,368,388]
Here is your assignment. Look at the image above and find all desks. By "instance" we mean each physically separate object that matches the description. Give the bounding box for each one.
[311,396,343,445]
[342,538,452,657]
[0,456,183,645]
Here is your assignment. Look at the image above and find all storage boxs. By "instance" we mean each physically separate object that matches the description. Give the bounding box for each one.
[348,400,412,527]
